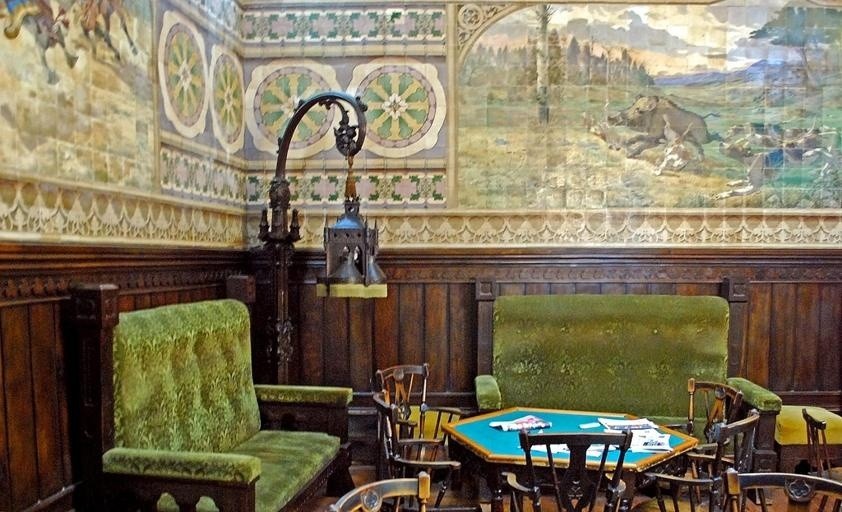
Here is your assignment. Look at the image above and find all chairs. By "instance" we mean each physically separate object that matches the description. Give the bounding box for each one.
[647,409,760,511]
[499,420,636,512]
[369,391,461,508]
[375,363,459,444]
[682,375,745,451]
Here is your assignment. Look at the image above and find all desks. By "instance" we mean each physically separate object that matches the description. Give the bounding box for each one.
[442,405,698,512]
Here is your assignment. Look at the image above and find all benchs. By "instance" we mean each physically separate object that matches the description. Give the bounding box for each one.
[474,276,782,505]
[72,275,351,512]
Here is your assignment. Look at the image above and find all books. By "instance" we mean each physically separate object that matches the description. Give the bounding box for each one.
[597,415,659,430]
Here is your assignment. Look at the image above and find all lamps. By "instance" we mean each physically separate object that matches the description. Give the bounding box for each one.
[316,156,389,299]
